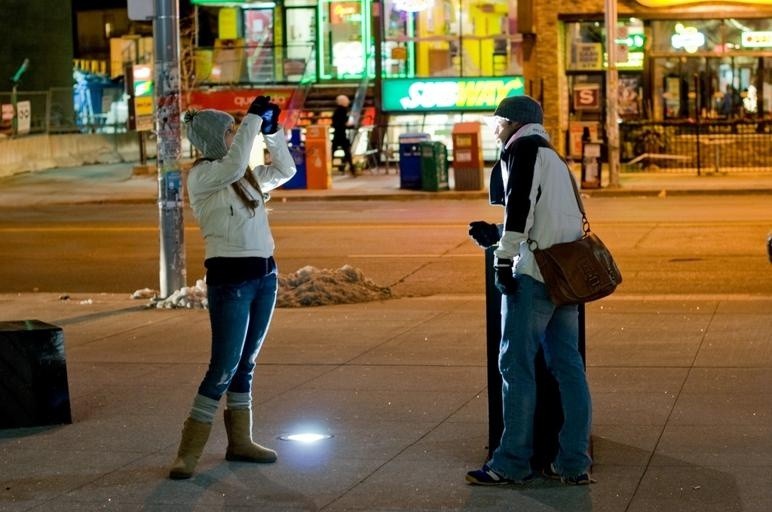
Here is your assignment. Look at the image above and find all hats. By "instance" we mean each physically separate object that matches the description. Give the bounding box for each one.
[186,108,236,160]
[494,95,544,125]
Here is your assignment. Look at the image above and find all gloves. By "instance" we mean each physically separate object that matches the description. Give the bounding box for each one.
[493,264,521,296]
[247,96,271,117]
[468,220,502,249]
[260,102,281,135]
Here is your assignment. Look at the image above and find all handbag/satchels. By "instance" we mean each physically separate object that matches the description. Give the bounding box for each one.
[533,227,623,308]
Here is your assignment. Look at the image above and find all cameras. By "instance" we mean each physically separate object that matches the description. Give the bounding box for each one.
[262,104,278,126]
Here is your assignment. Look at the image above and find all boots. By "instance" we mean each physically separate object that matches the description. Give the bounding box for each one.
[169,416,214,480]
[223,408,278,464]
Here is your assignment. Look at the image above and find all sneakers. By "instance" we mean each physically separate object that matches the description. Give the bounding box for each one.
[465,464,534,486]
[542,463,592,486]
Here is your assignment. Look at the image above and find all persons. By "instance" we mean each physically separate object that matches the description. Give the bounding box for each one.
[461,93,596,491]
[165,93,300,481]
[326,92,358,178]
